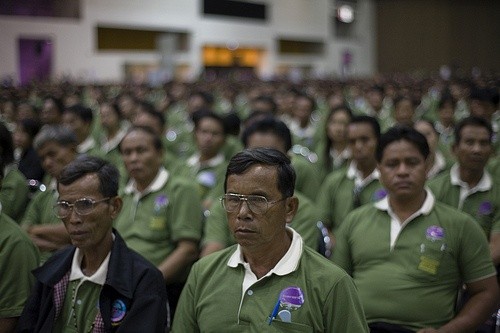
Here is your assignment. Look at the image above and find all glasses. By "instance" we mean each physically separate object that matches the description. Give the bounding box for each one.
[53,194,110,219]
[219,194,287,216]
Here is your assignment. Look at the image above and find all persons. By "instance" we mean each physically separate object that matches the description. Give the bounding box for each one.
[18,156,169,333]
[0,73,500,333]
[168,146,369,333]
[331,126,500,333]
